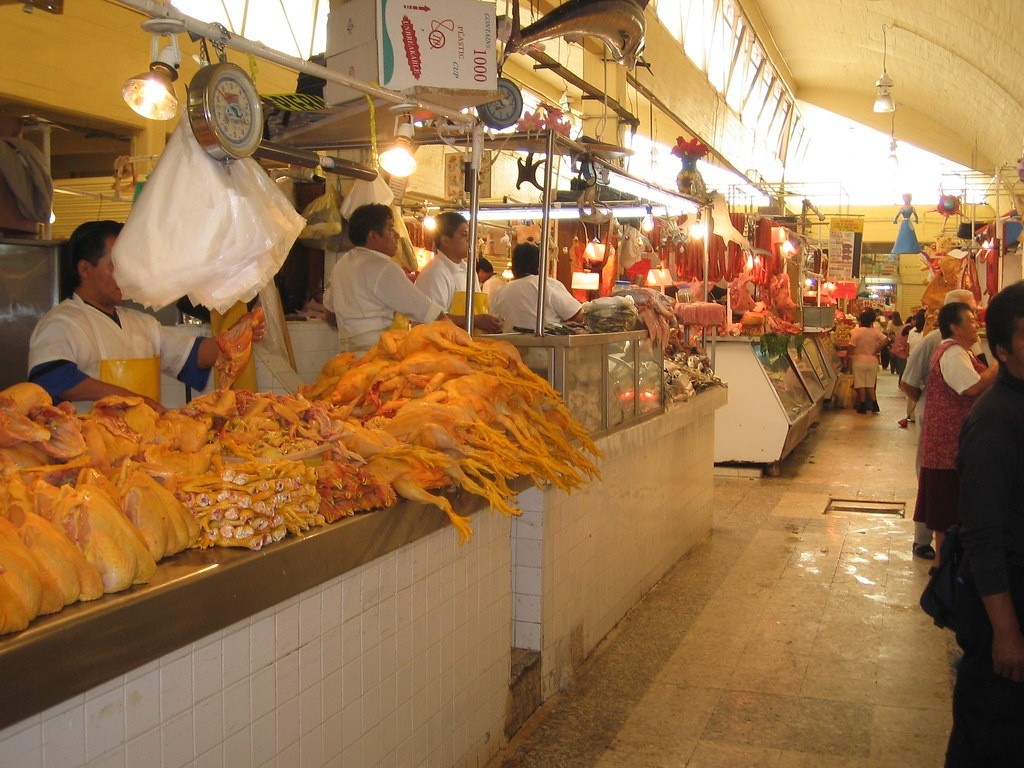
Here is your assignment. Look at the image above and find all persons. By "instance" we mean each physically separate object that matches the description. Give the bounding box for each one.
[321,203,446,361]
[890,193,921,254]
[475,257,503,308]
[414,210,504,335]
[847,307,927,414]
[492,241,584,334]
[26,220,265,415]
[901,280,1024,768]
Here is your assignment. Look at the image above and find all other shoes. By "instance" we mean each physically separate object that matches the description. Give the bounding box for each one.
[912,542,935,559]
[872,406,879,412]
[857,407,866,414]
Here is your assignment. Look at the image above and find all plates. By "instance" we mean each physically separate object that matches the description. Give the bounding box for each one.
[479,331,522,336]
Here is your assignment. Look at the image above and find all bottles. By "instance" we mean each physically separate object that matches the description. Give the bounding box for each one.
[612,280,632,293]
[677,285,693,303]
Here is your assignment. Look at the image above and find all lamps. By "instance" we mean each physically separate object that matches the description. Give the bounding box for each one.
[379,113,420,177]
[873,75,897,113]
[122,20,182,120]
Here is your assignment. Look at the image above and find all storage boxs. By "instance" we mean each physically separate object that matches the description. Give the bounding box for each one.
[323,0,496,105]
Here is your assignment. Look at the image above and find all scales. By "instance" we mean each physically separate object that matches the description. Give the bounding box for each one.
[186,22,265,171]
[475,60,523,130]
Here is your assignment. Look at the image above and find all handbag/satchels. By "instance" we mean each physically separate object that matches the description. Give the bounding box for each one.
[921,546,1024,661]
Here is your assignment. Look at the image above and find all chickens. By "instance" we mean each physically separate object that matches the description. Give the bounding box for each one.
[286,311,602,543]
[0,383,218,641]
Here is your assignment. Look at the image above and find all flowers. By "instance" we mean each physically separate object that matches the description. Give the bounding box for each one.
[672,135,708,163]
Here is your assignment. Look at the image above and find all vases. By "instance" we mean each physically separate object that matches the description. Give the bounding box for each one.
[677,158,701,194]
